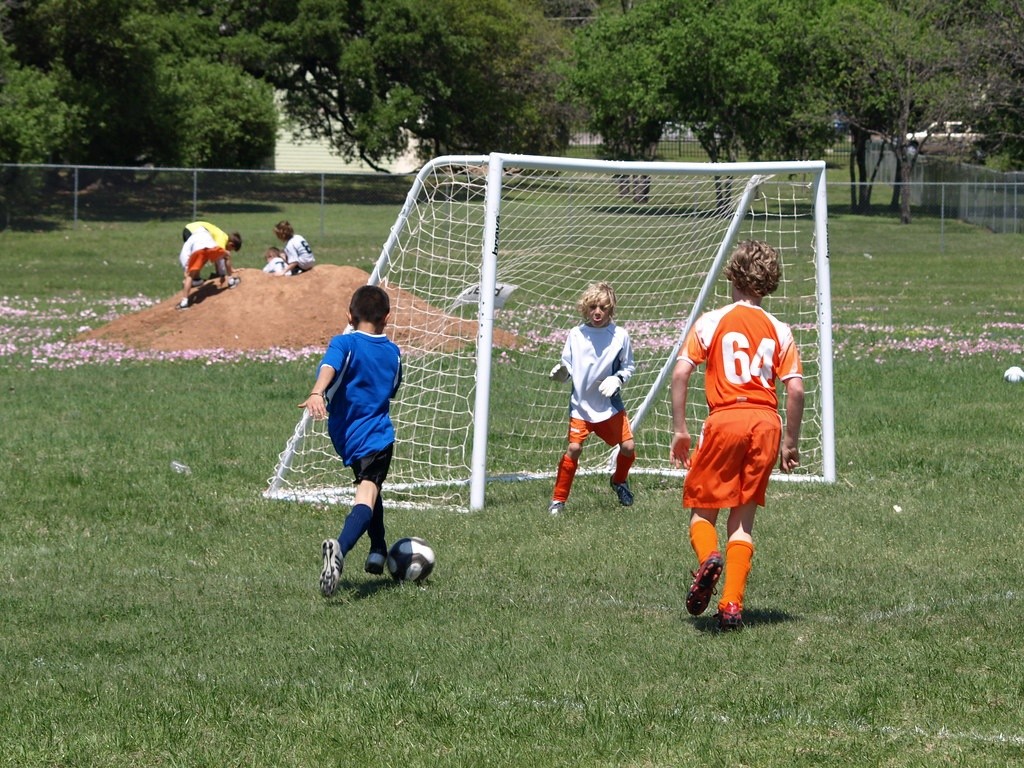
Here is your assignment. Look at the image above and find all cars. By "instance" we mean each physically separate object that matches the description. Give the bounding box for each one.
[903,121,984,160]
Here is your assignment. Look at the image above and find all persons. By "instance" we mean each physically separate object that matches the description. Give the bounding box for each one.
[548,282,637,517]
[670,239,805,631]
[175,221,314,309]
[297,287,402,596]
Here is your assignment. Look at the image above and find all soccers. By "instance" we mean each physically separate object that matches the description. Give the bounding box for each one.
[382,533,439,587]
[1002,366,1024,384]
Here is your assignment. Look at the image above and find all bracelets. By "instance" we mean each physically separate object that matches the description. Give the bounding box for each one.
[309,392,323,397]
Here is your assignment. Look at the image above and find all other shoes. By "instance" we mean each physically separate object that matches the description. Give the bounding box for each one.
[174,303,189,311]
[191,277,205,287]
[229,276,241,289]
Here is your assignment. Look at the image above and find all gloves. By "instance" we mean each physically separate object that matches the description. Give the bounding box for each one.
[598,376,623,398]
[548,364,569,383]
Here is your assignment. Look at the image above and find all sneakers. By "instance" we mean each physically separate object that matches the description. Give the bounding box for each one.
[319,538,345,598]
[364,549,388,575]
[549,500,565,515]
[609,475,634,506]
[711,601,742,630]
[685,552,723,615]
